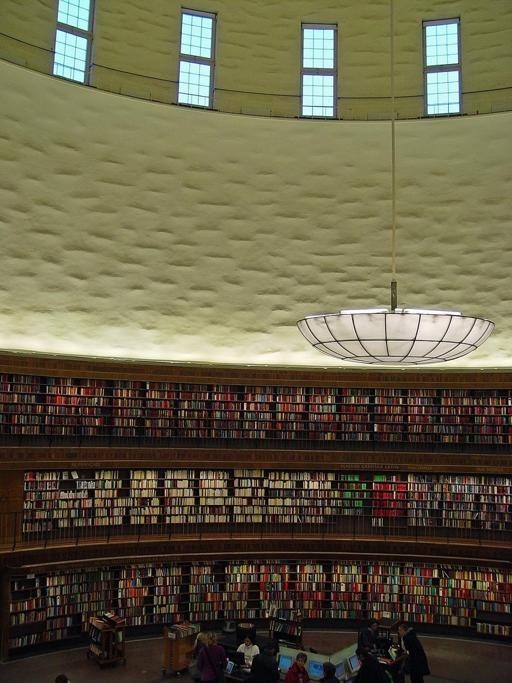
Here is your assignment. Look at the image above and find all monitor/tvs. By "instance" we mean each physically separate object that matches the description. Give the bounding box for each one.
[228,651,245,665]
[334,660,345,680]
[278,654,293,674]
[307,659,324,680]
[387,645,397,662]
[347,653,360,674]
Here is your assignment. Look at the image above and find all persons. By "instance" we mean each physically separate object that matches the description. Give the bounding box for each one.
[54,674,70,683]
[190,618,431,683]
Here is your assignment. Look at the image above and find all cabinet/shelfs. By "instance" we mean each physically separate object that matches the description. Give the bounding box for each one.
[0,350,511,659]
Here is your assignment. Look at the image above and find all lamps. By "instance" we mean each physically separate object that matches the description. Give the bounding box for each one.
[297,0,495,364]
